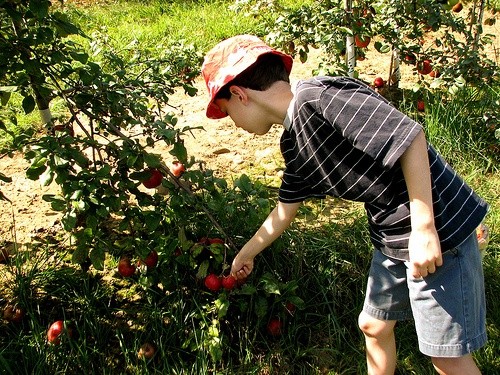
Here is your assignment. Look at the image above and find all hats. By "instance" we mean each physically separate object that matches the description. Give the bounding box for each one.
[201,35,293,119]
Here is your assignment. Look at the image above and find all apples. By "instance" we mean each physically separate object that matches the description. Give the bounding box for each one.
[282,301,294,314]
[355,8,441,111]
[143,168,163,188]
[46,321,72,344]
[269,320,281,334]
[172,163,184,177]
[118,237,247,289]
[3,305,21,321]
[452,3,463,12]
[140,343,154,357]
